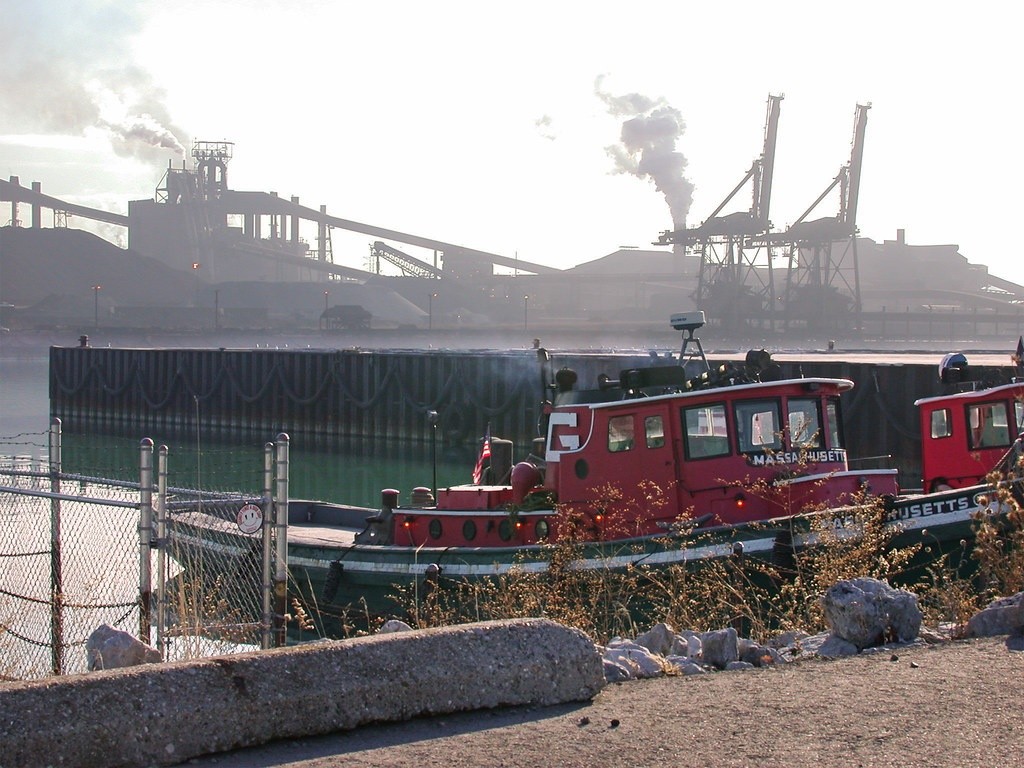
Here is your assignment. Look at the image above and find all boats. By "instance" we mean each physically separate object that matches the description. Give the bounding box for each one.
[139,311,1024,645]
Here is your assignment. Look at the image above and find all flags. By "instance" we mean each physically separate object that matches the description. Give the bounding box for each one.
[473,423,490,485]
